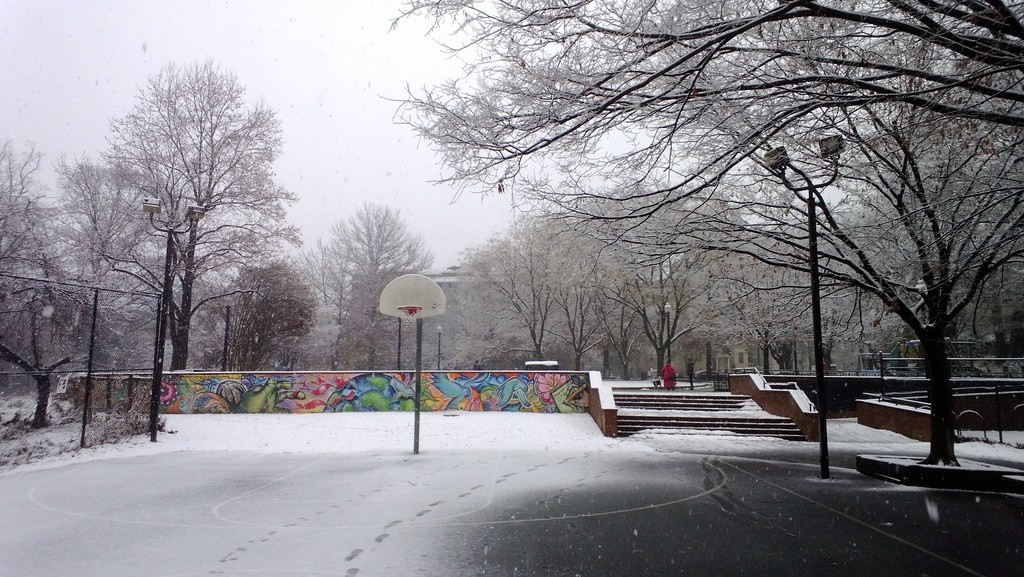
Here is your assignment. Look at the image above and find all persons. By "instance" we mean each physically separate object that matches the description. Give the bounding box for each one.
[686,355,696,391]
[660,359,677,392]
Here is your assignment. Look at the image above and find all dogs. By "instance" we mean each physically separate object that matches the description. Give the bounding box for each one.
[653,379,661,390]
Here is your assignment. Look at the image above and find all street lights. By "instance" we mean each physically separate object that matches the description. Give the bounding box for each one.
[436,325,442,371]
[665,301,672,365]
[141,197,206,442]
[220,295,233,372]
[765,132,844,479]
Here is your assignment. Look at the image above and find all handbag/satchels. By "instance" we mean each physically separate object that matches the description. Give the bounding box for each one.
[670,373,676,381]
